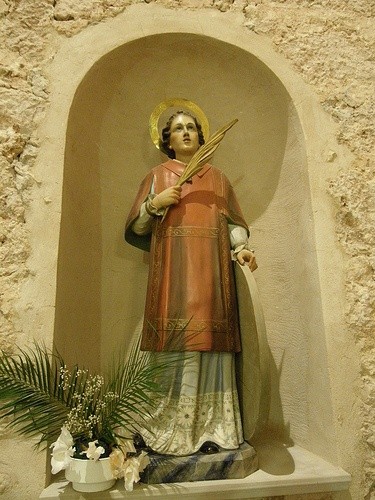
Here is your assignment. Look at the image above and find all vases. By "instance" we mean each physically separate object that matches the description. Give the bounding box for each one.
[64,456,118,493]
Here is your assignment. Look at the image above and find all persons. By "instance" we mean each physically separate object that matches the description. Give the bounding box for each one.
[124,112,258,454]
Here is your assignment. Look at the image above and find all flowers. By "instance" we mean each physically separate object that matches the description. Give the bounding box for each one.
[47,363,151,493]
